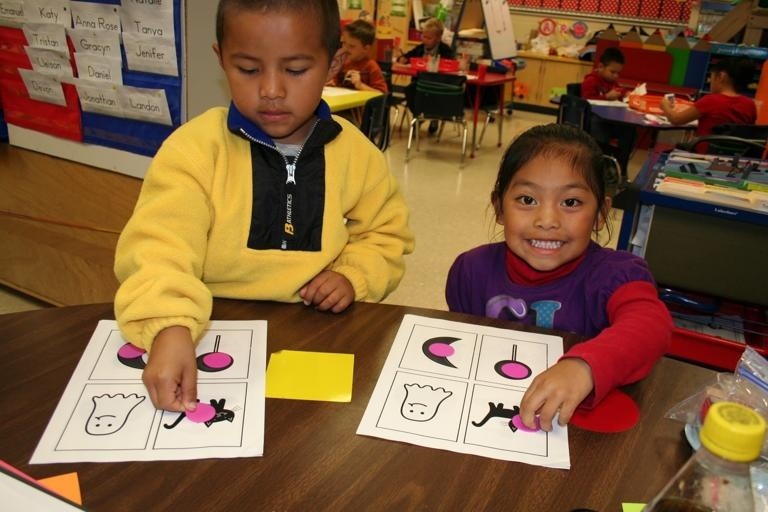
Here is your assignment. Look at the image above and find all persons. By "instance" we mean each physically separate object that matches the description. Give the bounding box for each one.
[114,0,415,411]
[397,17,457,136]
[581,46,631,101]
[326,19,387,93]
[445,124,674,431]
[661,53,757,154]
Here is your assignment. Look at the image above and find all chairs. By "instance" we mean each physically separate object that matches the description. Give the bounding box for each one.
[405,70,469,169]
[383,72,407,140]
[359,93,390,153]
[465,84,501,151]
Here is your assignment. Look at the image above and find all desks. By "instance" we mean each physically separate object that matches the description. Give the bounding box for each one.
[325,80,384,112]
[393,62,517,159]
[550,91,699,131]
[1,299,720,512]
[514,52,595,110]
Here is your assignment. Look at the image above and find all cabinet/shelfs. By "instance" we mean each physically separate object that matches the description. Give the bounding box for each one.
[617,149,768,307]
[0,121,144,308]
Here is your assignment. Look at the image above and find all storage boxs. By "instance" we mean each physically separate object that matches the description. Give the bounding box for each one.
[662,287,768,372]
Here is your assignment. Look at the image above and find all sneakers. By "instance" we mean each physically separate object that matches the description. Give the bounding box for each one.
[429,120,438,133]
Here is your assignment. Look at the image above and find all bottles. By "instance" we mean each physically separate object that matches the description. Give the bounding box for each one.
[638,400,767,512]
